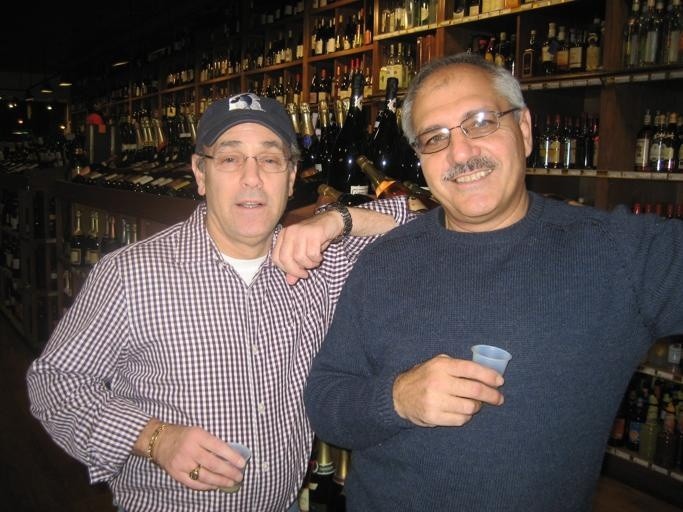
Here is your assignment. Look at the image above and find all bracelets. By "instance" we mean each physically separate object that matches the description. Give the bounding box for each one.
[148,423,172,459]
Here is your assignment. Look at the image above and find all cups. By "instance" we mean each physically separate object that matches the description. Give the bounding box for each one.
[469,344,513,391]
[218,439,250,494]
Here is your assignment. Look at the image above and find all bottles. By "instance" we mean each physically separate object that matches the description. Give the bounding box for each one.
[609,335,682,471]
[0,204,67,341]
[68,209,136,270]
[299,431,348,511]
[51,0,682,217]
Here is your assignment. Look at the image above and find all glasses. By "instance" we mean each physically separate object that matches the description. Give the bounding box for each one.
[195,148,294,174]
[409,105,520,155]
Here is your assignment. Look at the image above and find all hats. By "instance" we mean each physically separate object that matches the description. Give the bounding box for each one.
[194,92,297,154]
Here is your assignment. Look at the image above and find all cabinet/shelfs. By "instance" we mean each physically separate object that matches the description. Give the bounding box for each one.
[304,0,596,219]
[73,0,306,316]
[597,2,683,507]
[0,78,72,354]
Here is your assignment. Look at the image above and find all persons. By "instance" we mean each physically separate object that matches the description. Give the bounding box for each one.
[303,51,683,512]
[26,93,428,512]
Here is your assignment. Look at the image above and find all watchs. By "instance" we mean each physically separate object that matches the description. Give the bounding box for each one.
[314,201,353,244]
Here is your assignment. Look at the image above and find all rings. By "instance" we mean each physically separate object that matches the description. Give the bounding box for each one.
[188,465,200,480]
